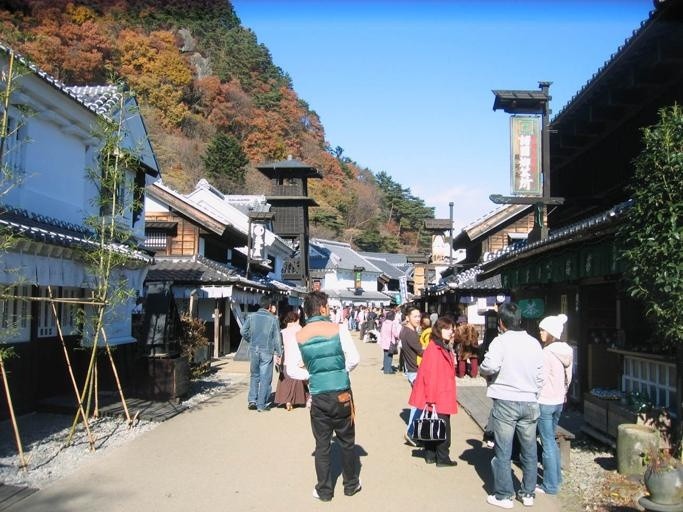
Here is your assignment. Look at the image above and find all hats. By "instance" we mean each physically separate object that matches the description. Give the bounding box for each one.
[456,316,468,323]
[539,314,568,339]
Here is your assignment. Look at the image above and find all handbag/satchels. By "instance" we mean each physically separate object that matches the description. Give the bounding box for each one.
[412,403,447,440]
[388,324,398,355]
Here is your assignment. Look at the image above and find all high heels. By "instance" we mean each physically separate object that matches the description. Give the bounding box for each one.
[286,403,292,411]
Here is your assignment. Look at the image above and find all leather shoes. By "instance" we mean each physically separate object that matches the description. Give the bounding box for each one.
[425,458,437,464]
[436,461,458,468]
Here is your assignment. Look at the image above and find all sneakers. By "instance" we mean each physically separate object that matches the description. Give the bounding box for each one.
[517,490,534,507]
[535,485,553,494]
[312,487,332,502]
[347,484,361,496]
[488,495,514,509]
[248,402,257,410]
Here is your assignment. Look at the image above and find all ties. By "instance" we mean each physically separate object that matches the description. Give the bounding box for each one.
[364,313,366,321]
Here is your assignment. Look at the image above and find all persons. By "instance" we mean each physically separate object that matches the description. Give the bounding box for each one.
[272,311,309,412]
[455,316,479,379]
[535,313,574,495]
[270,304,279,319]
[240,295,282,412]
[408,316,458,467]
[379,310,397,375]
[399,307,424,447]
[417,318,434,367]
[478,303,543,508]
[295,290,361,502]
[332,304,385,330]
